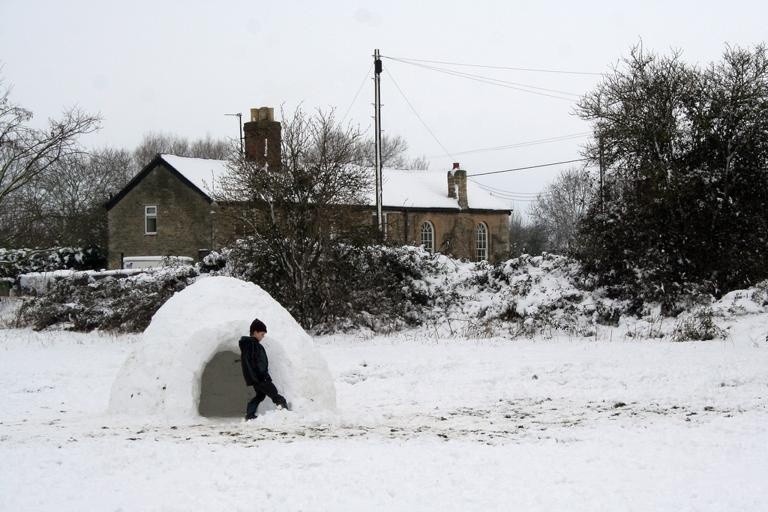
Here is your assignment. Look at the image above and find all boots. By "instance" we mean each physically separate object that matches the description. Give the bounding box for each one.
[246,403,257,420]
[277,399,289,410]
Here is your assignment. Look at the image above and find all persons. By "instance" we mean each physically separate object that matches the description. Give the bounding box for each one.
[239,317,288,420]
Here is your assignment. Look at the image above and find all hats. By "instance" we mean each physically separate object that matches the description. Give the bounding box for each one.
[250,319,267,333]
